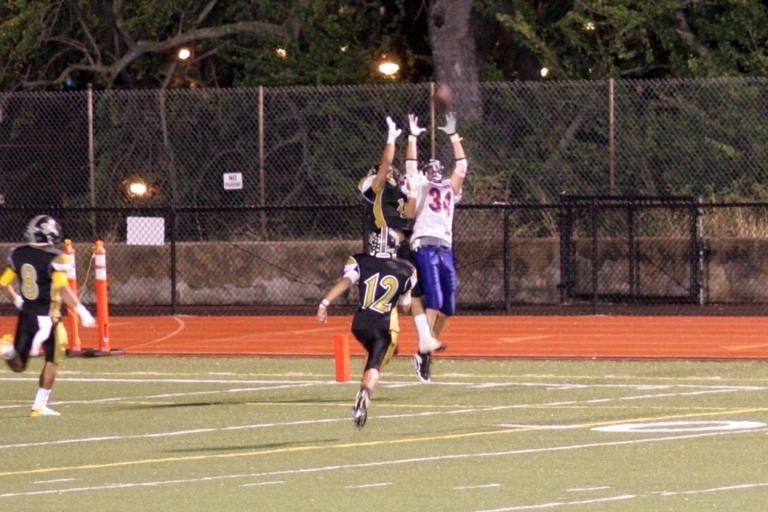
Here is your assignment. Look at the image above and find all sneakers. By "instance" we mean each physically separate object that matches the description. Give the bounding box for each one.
[417,338,445,356]
[411,350,432,381]
[30,402,59,416]
[352,386,370,431]
[0,333,15,358]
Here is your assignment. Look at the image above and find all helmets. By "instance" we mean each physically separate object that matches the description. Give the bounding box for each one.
[25,215,65,248]
[359,156,444,258]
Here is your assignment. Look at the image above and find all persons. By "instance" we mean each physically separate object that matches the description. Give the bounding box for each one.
[357,115,447,356]
[316,226,420,429]
[0,214,97,418]
[405,110,469,385]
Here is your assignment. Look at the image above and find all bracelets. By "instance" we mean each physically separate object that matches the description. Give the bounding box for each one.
[72,304,87,316]
[449,133,462,144]
[405,135,417,143]
[12,295,24,308]
[318,298,331,309]
[408,190,419,199]
[385,136,396,145]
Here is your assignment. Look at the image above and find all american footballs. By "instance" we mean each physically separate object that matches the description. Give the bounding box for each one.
[433,83,452,109]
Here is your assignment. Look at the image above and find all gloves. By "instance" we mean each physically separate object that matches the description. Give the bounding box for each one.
[385,112,460,145]
[75,303,97,329]
